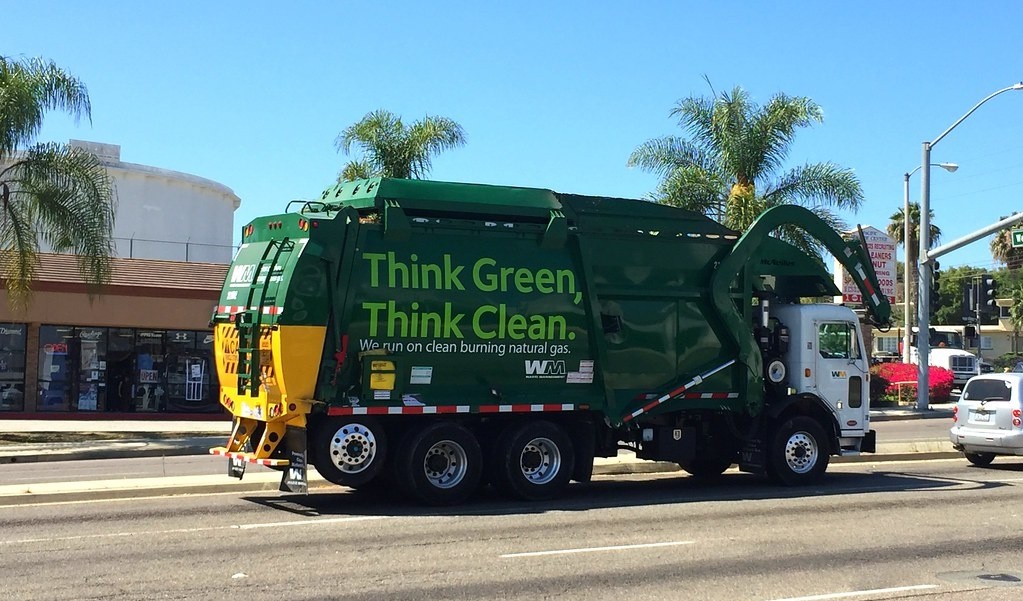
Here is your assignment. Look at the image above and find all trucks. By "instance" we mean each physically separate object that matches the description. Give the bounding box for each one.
[872,324,979,393]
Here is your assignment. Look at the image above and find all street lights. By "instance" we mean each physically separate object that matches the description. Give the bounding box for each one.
[902,163,959,364]
[916,82,1023,412]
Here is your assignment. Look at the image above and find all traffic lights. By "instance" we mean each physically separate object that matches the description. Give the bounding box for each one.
[981,274,997,312]
[962,327,975,338]
[929,257,940,316]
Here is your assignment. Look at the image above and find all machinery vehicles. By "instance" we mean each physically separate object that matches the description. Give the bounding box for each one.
[208,177,892,508]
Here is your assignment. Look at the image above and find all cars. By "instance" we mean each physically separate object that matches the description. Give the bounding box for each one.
[950,373,1023,467]
[2,382,65,411]
